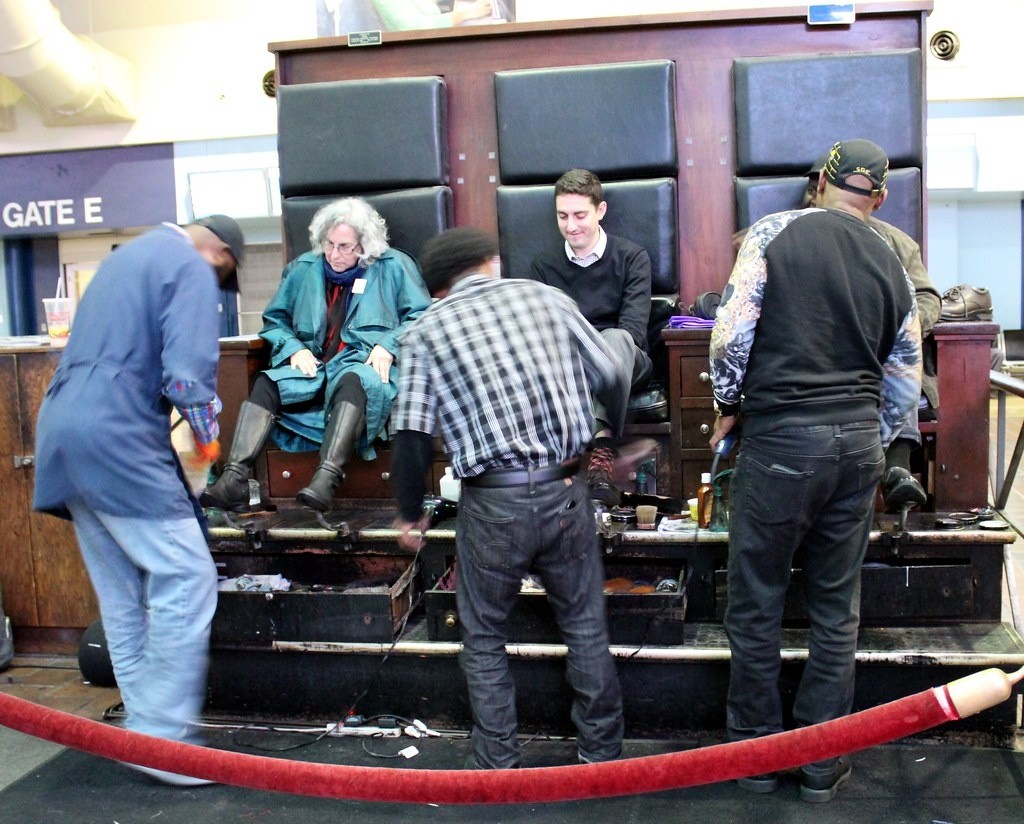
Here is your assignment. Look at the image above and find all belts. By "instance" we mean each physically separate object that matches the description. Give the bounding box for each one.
[466,460,581,490]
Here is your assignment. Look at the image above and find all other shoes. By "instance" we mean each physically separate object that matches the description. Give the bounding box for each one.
[739,771,776,793]
[882,468,927,514]
[798,753,852,802]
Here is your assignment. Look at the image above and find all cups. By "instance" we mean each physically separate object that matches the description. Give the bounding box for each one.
[42,298,72,341]
[687,497,698,522]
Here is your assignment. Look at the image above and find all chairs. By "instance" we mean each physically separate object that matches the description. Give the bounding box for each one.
[216,76,453,497]
[491,58,681,507]
[735,49,1001,516]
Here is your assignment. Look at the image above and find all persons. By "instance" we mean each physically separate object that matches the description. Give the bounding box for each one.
[800,157,941,516]
[528,168,653,505]
[388,225,622,770]
[710,138,923,803]
[31,214,246,785]
[199,197,433,518]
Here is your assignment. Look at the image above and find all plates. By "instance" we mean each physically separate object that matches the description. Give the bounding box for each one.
[934,510,1010,529]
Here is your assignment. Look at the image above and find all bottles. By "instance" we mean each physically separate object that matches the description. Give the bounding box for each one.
[697,472,713,529]
[438,466,462,502]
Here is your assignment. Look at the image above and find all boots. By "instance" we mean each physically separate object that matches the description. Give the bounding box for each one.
[296,402,368,511]
[195,401,272,513]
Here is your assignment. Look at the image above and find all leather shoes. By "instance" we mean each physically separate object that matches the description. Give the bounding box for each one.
[588,469,619,505]
[678,292,723,319]
[938,287,994,321]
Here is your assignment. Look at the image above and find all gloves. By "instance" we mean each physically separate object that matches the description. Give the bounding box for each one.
[182,392,221,466]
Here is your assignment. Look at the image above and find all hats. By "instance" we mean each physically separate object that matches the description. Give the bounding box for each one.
[806,156,829,173]
[197,215,242,294]
[827,138,889,197]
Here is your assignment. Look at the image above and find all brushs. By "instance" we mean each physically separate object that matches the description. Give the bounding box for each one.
[635,505,658,529]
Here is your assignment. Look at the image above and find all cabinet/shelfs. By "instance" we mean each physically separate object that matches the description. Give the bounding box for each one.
[660,327,739,511]
[0,346,105,655]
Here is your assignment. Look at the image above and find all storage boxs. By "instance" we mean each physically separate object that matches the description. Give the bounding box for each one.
[423,561,687,650]
[192,559,419,642]
[713,557,977,633]
[268,444,433,500]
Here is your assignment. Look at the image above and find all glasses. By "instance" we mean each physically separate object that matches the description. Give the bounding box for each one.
[319,238,359,256]
